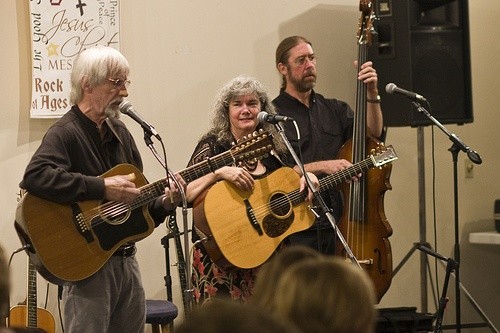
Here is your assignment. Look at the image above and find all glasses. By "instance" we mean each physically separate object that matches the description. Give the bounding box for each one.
[106,77,131,89]
[285,55,319,64]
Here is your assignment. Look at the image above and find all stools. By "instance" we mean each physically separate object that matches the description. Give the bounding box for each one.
[146,300,178,333]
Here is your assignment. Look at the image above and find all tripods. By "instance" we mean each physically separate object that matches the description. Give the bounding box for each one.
[392,128,500,333]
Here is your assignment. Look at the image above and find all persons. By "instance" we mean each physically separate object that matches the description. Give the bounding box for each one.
[178,244,377,333]
[176,78,319,308]
[0,244,51,333]
[271,36,387,255]
[17,36,187,333]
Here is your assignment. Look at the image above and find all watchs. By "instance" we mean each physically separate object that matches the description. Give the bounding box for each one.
[366,95,381,102]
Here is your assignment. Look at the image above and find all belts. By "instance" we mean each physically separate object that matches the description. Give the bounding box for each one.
[113,244,137,257]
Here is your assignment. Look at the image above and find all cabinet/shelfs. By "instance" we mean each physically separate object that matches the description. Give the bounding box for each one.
[362,0,475,128]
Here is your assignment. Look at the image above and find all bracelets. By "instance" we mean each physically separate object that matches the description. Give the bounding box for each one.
[162,197,167,203]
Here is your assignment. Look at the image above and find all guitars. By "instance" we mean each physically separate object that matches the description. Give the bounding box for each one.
[13,128,277,287]
[192,142,400,274]
[166,209,190,314]
[6,190,57,333]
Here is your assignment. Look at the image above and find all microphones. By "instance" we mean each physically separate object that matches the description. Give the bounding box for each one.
[257,112,293,124]
[119,101,162,141]
[386,83,426,103]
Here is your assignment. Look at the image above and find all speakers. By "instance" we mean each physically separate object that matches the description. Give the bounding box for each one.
[364,0,474,128]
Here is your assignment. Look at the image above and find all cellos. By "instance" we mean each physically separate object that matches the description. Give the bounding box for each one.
[333,0,395,306]
